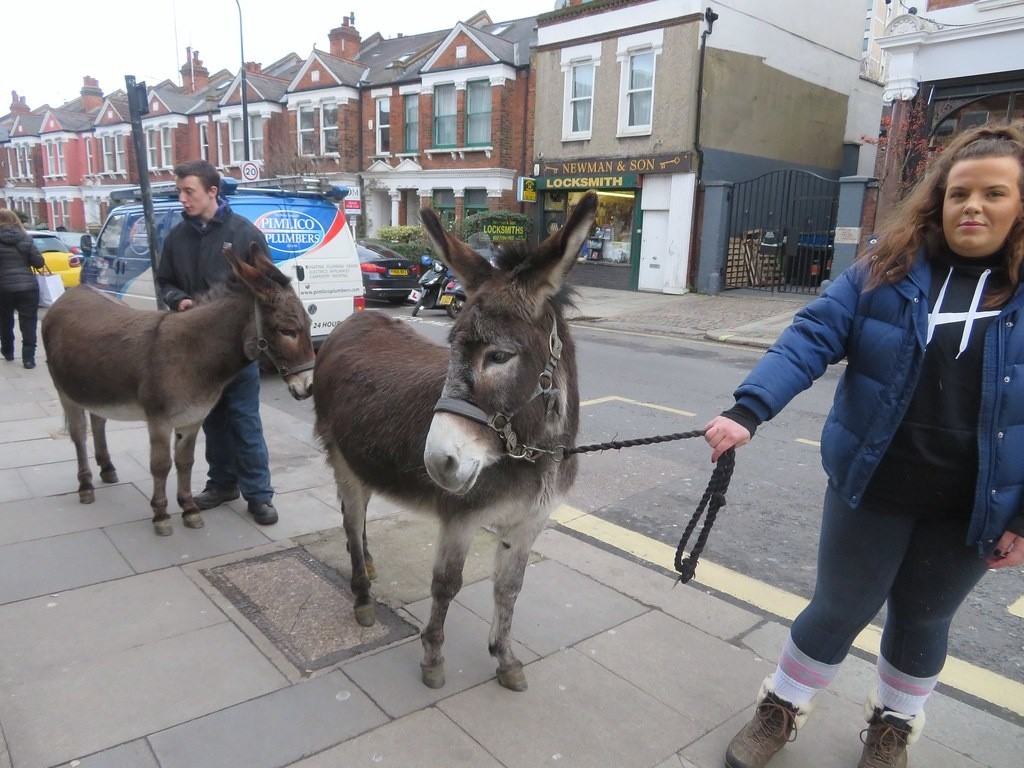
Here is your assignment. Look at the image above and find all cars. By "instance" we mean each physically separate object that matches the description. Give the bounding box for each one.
[24,231,83,305]
[353,240,422,307]
[56,231,97,267]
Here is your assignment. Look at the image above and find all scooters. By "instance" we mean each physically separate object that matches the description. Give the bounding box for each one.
[439,268,468,319]
[412,245,454,317]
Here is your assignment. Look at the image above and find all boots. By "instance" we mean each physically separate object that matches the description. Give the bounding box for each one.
[725,674,815,768]
[858,686,926,768]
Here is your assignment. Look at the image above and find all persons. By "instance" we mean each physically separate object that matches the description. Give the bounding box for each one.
[155,160,278,525]
[0,207,45,369]
[702,117,1024,767]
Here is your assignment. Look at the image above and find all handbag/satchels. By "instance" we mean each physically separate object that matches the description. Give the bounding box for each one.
[32,264,65,306]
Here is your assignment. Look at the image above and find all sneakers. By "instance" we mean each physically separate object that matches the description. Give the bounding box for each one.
[192,487,241,508]
[247,501,278,525]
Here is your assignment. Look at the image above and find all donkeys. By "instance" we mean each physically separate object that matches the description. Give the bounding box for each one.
[313,190,599,691]
[41,241,317,536]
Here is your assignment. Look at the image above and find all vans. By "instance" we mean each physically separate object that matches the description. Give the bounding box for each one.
[74,175,366,377]
